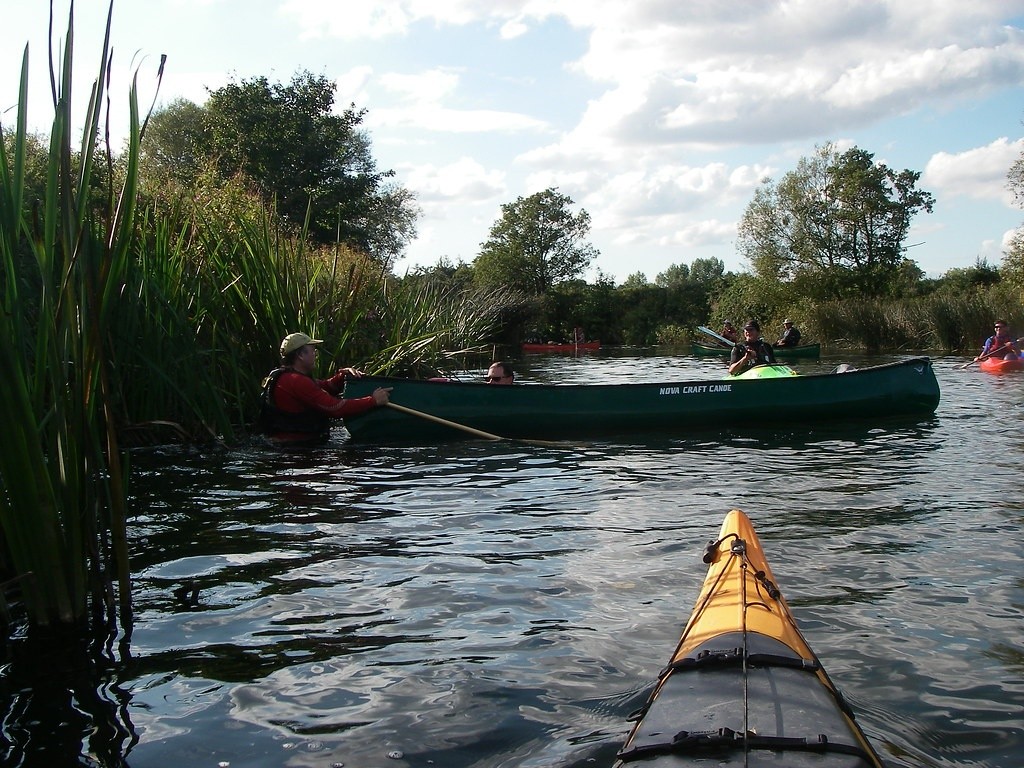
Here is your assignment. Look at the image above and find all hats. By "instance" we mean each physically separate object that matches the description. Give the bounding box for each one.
[744,319,759,332]
[279,333,324,360]
[784,319,792,324]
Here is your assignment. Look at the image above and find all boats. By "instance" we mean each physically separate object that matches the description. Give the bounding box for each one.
[520,339,603,354]
[718,364,798,382]
[689,340,824,360]
[979,349,1024,372]
[340,354,943,435]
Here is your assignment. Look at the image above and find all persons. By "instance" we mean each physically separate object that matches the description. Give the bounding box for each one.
[772,319,801,348]
[974,320,1021,362]
[570,327,585,344]
[729,320,776,375]
[719,319,737,347]
[487,361,514,385]
[260,332,393,439]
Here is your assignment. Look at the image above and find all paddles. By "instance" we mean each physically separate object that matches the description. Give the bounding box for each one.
[953,335,1023,370]
[733,342,772,364]
[380,384,522,445]
[573,328,578,357]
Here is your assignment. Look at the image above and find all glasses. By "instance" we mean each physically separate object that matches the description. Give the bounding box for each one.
[484,376,507,382]
[995,325,1004,328]
